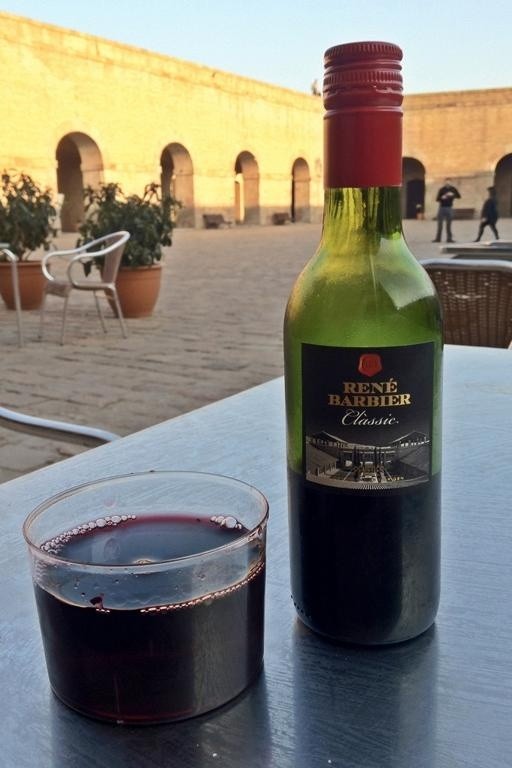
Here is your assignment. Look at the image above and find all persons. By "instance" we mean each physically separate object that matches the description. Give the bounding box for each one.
[431,177,461,243]
[472,185,498,243]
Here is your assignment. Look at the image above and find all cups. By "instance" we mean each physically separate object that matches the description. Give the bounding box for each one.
[25,470,268,726]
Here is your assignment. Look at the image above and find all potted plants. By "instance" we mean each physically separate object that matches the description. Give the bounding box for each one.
[0,170,57,310]
[77,182,182,319]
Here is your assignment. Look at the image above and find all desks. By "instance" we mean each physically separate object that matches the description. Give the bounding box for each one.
[1,343,512,762]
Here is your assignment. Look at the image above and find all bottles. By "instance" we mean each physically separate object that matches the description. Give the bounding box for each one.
[281,41,442,647]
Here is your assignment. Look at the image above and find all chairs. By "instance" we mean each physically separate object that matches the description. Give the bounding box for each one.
[39,230,132,345]
[417,257,512,351]
